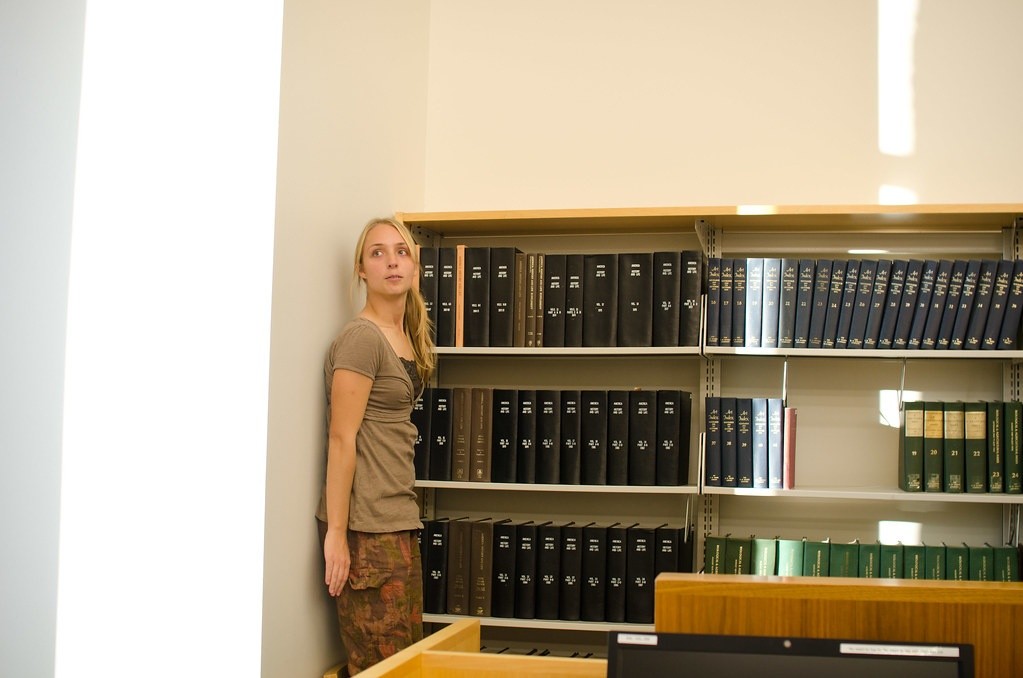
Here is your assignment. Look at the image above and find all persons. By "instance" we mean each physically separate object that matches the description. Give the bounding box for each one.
[315,217,438,676]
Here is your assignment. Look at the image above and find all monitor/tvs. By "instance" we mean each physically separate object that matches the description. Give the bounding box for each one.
[606,631,976,678]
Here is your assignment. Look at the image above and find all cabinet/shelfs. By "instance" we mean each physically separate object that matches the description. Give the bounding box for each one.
[390,202,1023,662]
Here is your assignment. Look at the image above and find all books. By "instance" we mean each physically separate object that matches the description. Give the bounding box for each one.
[707,258,1023,350]
[419,247,701,348]
[706,397,798,490]
[899,399,1023,495]
[409,385,692,486]
[706,533,1023,582]
[416,516,694,624]
